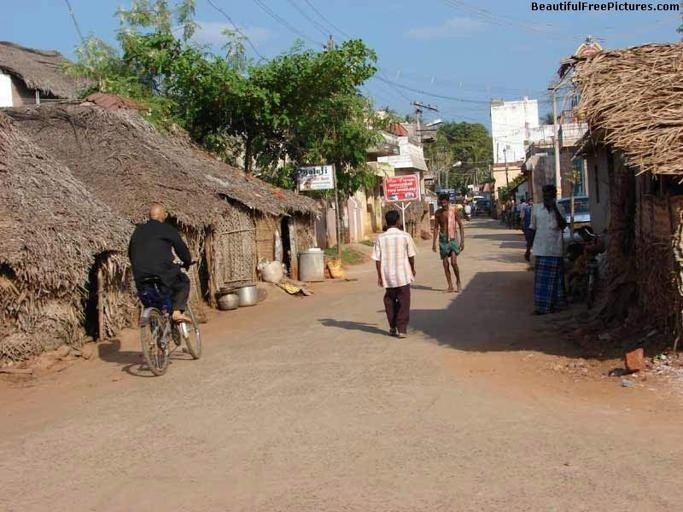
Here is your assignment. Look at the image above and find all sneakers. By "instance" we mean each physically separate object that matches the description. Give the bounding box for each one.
[389,327,407,338]
[172,313,191,323]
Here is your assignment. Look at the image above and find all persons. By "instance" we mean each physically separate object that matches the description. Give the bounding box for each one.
[128,203,192,351]
[491,195,535,246]
[450,198,479,221]
[524,184,568,314]
[432,193,465,292]
[372,209,417,338]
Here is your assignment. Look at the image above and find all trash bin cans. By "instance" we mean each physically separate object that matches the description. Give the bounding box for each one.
[298,247,325,282]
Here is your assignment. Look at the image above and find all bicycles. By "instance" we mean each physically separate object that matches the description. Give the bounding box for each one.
[136,260,203,375]
